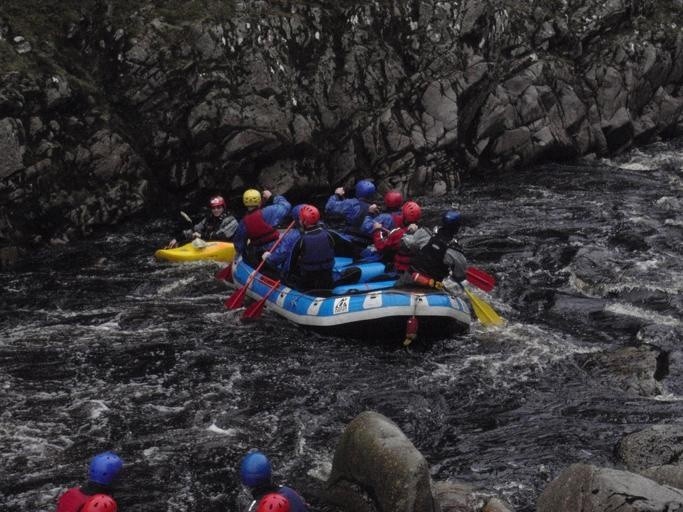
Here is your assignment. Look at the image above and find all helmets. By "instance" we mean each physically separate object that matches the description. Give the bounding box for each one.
[209,195,226,209]
[243,189,262,208]
[402,201,422,222]
[86,450,125,489]
[384,191,403,208]
[299,205,321,224]
[355,179,376,196]
[291,204,307,215]
[442,209,462,224]
[238,450,272,484]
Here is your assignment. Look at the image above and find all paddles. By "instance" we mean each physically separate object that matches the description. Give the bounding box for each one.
[370,219,495,291]
[216,253,241,279]
[180,210,206,248]
[226,218,295,309]
[245,278,281,318]
[453,275,504,326]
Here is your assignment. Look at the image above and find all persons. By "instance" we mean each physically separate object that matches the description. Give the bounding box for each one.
[373,200,423,273]
[80,493,118,512]
[233,189,293,272]
[256,492,291,512]
[394,209,467,293]
[239,452,307,512]
[323,180,376,257]
[279,205,353,293]
[167,194,239,249]
[261,204,310,282]
[55,451,124,512]
[360,191,403,257]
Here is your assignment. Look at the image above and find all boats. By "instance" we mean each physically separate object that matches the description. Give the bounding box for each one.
[230,247,472,337]
[154,240,235,265]
